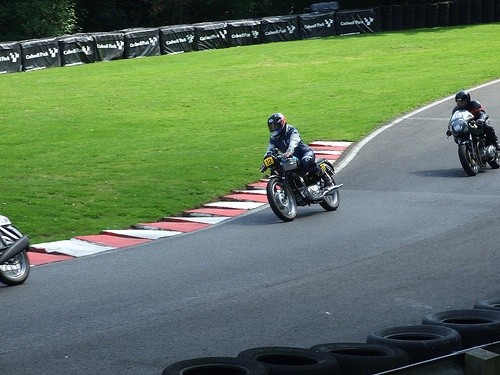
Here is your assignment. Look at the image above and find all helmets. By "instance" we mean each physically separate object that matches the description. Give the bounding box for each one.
[454,92,473,108]
[268,114,288,140]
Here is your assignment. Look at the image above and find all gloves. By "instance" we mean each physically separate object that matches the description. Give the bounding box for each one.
[280,151,298,161]
[476,117,490,128]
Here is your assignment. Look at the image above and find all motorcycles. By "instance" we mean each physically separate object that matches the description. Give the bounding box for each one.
[445,109,500,176]
[258,147,344,222]
[0,215,32,286]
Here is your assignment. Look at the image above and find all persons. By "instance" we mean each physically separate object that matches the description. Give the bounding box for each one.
[445,91,500,151]
[263,113,332,186]
